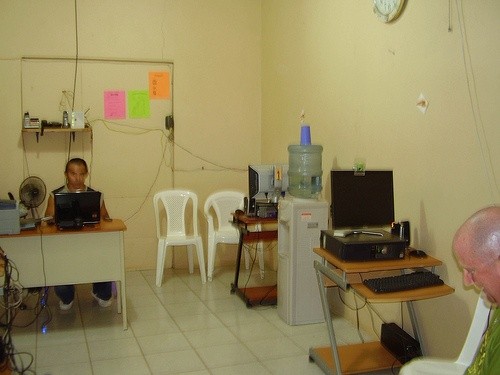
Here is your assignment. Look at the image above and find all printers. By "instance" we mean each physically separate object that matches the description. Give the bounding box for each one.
[0,199,22,235]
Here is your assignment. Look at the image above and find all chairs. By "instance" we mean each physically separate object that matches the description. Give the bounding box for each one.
[203,191,265,281]
[153,190,206,287]
[400,287,495,375]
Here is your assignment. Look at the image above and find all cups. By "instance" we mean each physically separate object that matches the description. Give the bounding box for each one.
[300,126,311,146]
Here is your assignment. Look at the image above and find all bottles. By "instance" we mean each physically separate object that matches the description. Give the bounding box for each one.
[287,145,324,198]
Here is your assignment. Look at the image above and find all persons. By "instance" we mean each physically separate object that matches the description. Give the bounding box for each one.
[451,206,500,375]
[44,158,113,309]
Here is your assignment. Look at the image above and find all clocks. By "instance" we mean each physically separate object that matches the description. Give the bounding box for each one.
[372,1,404,24]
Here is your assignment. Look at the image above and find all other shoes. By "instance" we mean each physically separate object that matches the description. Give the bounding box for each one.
[91,292,115,307]
[58,299,74,311]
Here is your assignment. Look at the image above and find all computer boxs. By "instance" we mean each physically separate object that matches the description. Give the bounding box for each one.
[319,230,408,262]
[243,197,278,218]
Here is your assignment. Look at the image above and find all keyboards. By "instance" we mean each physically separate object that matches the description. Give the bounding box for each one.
[364,272,444,294]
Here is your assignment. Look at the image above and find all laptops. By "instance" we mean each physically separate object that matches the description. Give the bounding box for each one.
[54,192,101,224]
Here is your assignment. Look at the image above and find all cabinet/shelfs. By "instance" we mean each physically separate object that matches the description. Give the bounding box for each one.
[309,248,455,375]
[229,213,278,309]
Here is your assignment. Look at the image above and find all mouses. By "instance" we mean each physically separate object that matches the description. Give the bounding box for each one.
[235,209,244,215]
[409,250,427,258]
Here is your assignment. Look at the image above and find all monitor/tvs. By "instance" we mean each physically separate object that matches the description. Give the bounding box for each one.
[330,170,395,238]
[249,164,289,200]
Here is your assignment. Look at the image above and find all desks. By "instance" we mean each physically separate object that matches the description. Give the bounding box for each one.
[0,218,128,331]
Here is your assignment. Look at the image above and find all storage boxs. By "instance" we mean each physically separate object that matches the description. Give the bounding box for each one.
[19,176,47,218]
